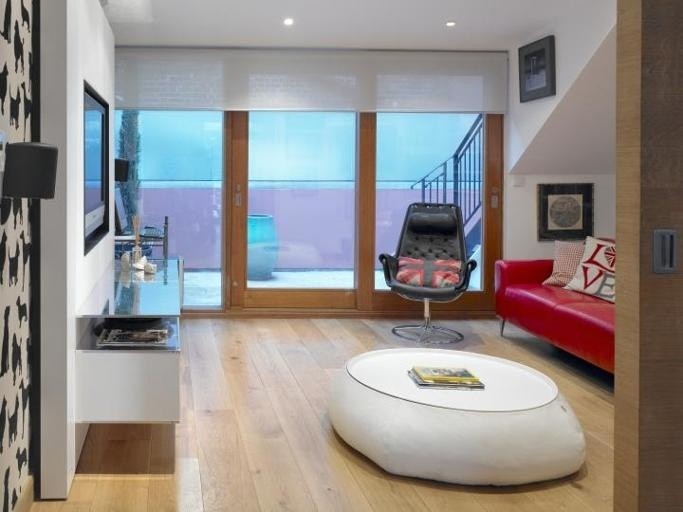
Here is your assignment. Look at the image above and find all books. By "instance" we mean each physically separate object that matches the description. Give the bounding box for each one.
[95,327,169,348]
[407,364,486,391]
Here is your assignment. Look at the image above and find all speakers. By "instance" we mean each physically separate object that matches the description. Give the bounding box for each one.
[110,158,130,184]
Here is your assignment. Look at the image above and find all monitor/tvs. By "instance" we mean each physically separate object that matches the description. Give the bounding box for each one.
[84,90,106,238]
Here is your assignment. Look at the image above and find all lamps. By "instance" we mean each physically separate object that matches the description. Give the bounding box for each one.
[115,158,129,182]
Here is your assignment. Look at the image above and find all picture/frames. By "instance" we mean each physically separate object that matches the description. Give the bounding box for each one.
[537,182,593,243]
[520,34,557,104]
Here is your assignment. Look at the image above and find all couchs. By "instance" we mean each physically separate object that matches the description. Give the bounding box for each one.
[492,259,615,377]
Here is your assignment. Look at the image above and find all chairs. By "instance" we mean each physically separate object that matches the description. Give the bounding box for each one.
[379,204,476,345]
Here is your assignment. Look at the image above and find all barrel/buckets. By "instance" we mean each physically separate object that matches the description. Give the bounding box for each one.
[248,213,278,280]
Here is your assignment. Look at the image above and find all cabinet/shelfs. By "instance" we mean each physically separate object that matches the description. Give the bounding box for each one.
[138,216,169,258]
[74,260,182,424]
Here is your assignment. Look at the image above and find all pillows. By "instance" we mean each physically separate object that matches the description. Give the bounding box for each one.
[569,236,614,303]
[396,255,463,288]
[542,239,585,286]
